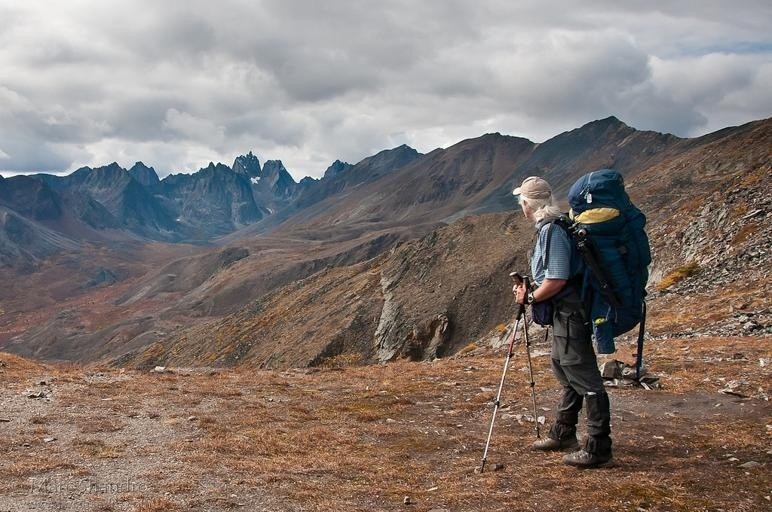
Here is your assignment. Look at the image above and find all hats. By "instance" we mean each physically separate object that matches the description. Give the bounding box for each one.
[513,176,550,200]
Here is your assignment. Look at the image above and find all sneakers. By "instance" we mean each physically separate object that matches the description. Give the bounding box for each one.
[534,436,579,451]
[562,449,614,468]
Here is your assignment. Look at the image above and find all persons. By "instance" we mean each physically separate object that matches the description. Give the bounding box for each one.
[511,176,612,467]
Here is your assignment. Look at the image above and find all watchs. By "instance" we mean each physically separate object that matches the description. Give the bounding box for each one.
[528,293,534,304]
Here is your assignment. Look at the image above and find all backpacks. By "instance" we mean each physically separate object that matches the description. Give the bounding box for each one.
[539,169,651,355]
